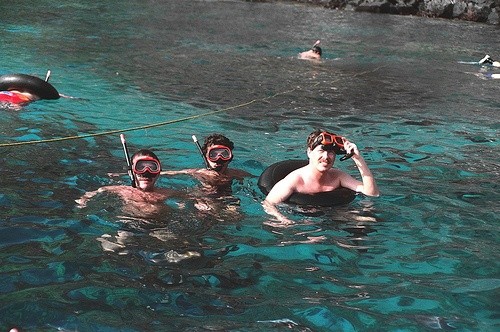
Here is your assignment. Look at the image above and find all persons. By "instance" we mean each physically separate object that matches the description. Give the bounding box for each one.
[263,130,380,227]
[478,55,500,80]
[297,46,322,61]
[75,149,240,260]
[107,135,258,219]
[0,75,80,113]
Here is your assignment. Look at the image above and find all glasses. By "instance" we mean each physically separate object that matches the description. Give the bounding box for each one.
[308,132,346,154]
[205,145,233,162]
[131,157,162,175]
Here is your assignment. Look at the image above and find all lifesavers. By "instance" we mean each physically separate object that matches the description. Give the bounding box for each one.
[1,72,60,100]
[256,157,360,209]
